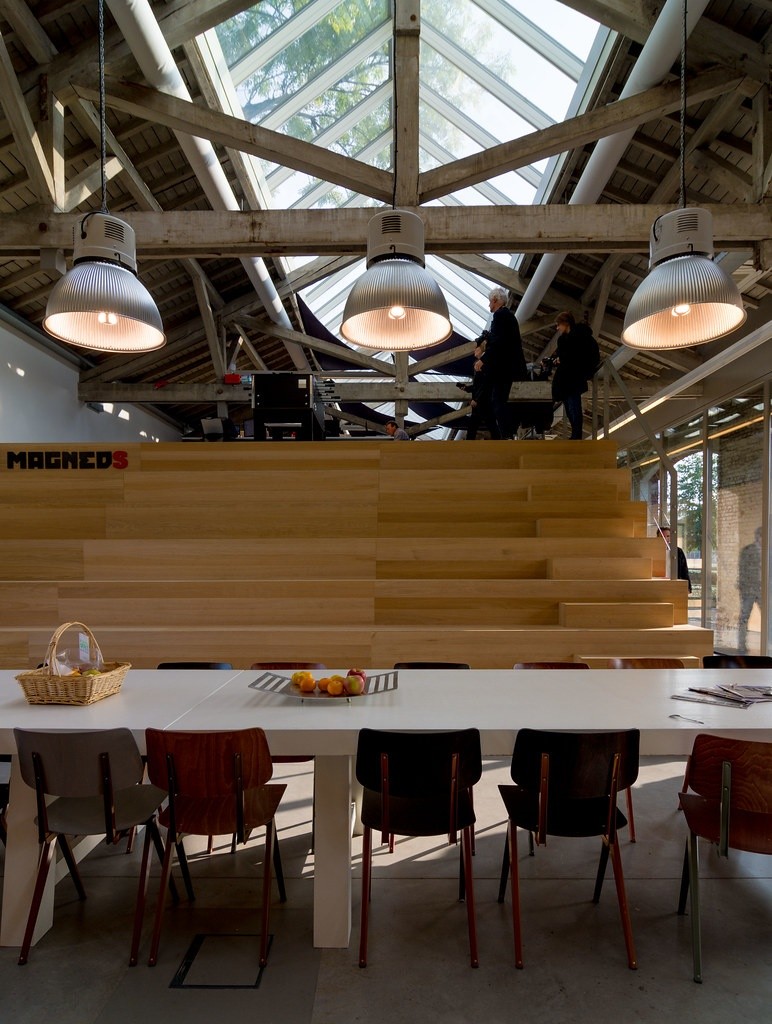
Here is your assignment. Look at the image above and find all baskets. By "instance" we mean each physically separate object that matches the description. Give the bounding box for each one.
[13,621,131,706]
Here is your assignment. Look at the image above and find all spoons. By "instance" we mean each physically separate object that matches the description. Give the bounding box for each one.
[669,714,704,724]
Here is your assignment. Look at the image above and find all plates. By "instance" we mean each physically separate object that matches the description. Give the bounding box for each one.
[247,671,398,698]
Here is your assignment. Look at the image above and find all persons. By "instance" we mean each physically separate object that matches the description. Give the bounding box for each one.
[386,421,410,441]
[540,312,600,440]
[733,526,762,654]
[656,527,693,596]
[458,287,529,441]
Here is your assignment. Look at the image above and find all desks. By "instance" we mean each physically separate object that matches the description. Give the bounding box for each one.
[0,667,772,946]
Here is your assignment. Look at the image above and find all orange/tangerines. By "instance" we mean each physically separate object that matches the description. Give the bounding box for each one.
[292,671,345,695]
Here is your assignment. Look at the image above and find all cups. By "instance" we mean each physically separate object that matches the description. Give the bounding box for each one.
[240,430,244,437]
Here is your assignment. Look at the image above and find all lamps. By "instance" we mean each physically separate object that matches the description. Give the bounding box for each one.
[335,1,455,353]
[620,0,750,352]
[44,1,171,353]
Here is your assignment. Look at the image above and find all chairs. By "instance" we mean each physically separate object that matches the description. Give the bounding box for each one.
[502,728,638,967]
[135,726,289,969]
[10,727,170,969]
[677,734,770,981]
[355,726,479,966]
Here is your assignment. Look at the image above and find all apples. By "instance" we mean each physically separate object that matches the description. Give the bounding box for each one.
[345,669,366,694]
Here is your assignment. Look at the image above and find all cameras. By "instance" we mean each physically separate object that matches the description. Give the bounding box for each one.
[542,357,556,366]
